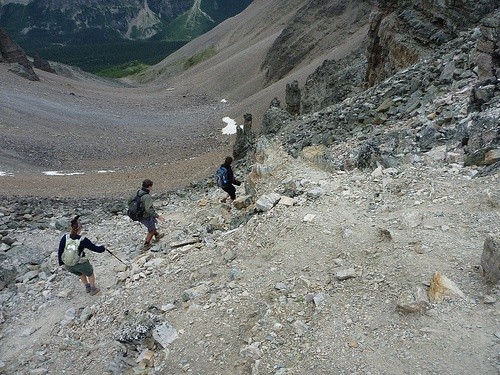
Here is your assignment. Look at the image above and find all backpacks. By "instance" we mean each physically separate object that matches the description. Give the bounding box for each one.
[126,190,148,220]
[61,234,86,266]
[215,165,229,188]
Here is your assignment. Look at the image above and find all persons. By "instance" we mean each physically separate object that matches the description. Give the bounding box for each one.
[132,179,165,250]
[221,157,241,200]
[58,220,106,296]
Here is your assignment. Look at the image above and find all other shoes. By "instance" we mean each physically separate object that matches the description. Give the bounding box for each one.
[220,199,226,203]
[156,234,164,241]
[143,244,152,250]
[86,288,91,293]
[90,288,99,296]
